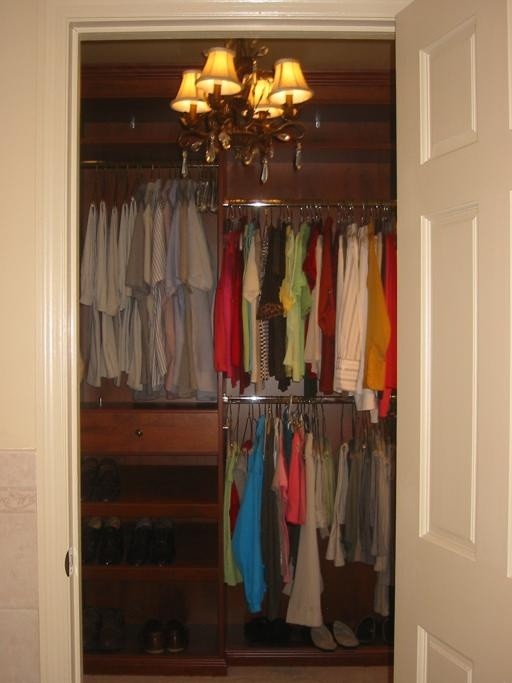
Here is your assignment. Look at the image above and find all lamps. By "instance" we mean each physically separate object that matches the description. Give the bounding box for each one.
[168,38,317,182]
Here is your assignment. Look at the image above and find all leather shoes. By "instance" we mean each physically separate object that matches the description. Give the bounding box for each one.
[79,457,99,503]
[82,606,185,653]
[82,514,175,568]
[97,457,121,504]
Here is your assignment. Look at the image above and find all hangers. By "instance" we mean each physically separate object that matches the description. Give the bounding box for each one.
[78,167,395,246]
[223,396,397,451]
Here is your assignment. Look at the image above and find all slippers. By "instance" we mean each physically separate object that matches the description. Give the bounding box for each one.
[244,612,395,649]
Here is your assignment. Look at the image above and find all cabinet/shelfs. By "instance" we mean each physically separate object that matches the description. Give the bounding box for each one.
[80,64,397,683]
